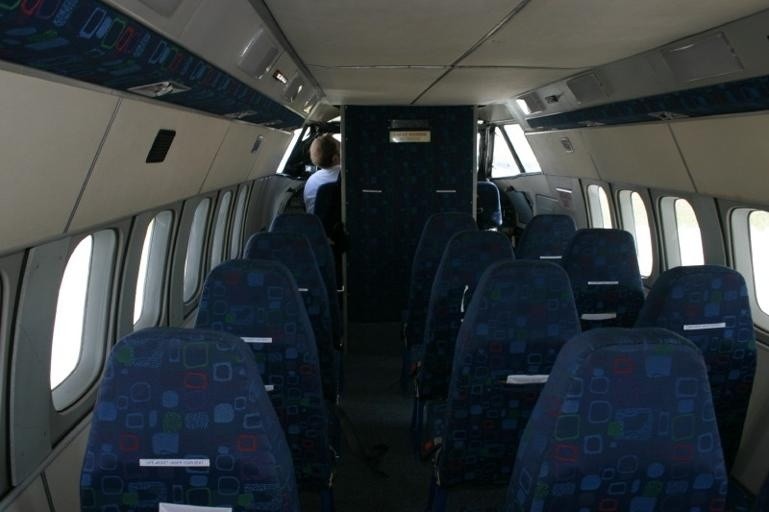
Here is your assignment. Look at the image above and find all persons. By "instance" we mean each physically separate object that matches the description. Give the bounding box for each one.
[302,135,341,216]
